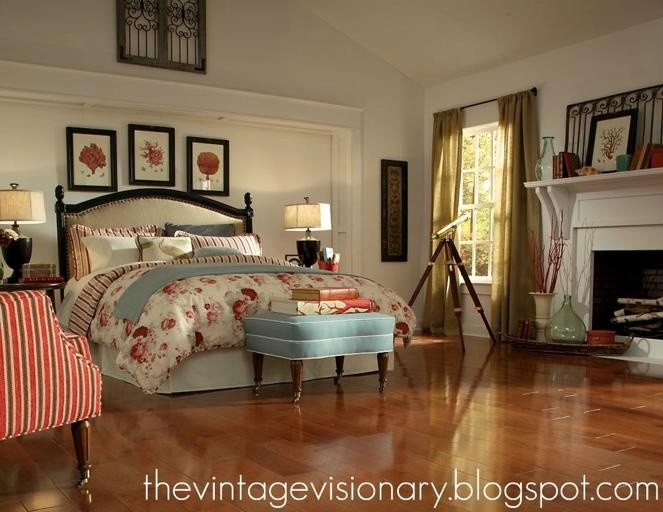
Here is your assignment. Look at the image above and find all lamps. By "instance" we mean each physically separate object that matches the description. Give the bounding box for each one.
[284,196,332,268]
[1,182,46,285]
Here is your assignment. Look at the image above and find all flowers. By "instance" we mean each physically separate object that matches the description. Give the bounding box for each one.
[526,205,566,293]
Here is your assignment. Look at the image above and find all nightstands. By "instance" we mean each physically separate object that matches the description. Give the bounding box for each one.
[1,282,66,314]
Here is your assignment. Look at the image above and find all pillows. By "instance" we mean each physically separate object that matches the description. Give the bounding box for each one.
[173,230,263,257]
[193,245,245,259]
[163,221,235,237]
[80,236,142,274]
[135,234,194,263]
[66,222,166,282]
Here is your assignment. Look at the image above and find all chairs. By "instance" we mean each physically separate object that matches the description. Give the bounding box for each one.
[0,288,103,486]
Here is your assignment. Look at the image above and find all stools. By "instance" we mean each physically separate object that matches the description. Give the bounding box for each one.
[244,314,395,404]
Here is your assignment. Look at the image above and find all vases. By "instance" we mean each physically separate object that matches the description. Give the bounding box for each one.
[534,135,558,181]
[528,291,558,344]
[544,291,587,342]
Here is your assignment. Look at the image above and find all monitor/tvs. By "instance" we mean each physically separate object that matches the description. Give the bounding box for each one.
[588,247,663,341]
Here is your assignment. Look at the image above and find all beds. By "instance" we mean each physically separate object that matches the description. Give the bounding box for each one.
[54,185,418,399]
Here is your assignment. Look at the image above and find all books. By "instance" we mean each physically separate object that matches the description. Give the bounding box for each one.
[268,286,379,316]
[629,142,655,170]
[552,151,579,179]
[22,276,64,282]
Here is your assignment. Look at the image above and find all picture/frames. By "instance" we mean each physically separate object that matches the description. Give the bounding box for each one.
[585,107,639,174]
[284,253,303,267]
[186,136,231,198]
[128,123,177,189]
[66,126,119,194]
[380,159,409,262]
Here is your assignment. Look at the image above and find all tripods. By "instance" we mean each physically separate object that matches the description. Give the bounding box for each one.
[408,229,497,354]
